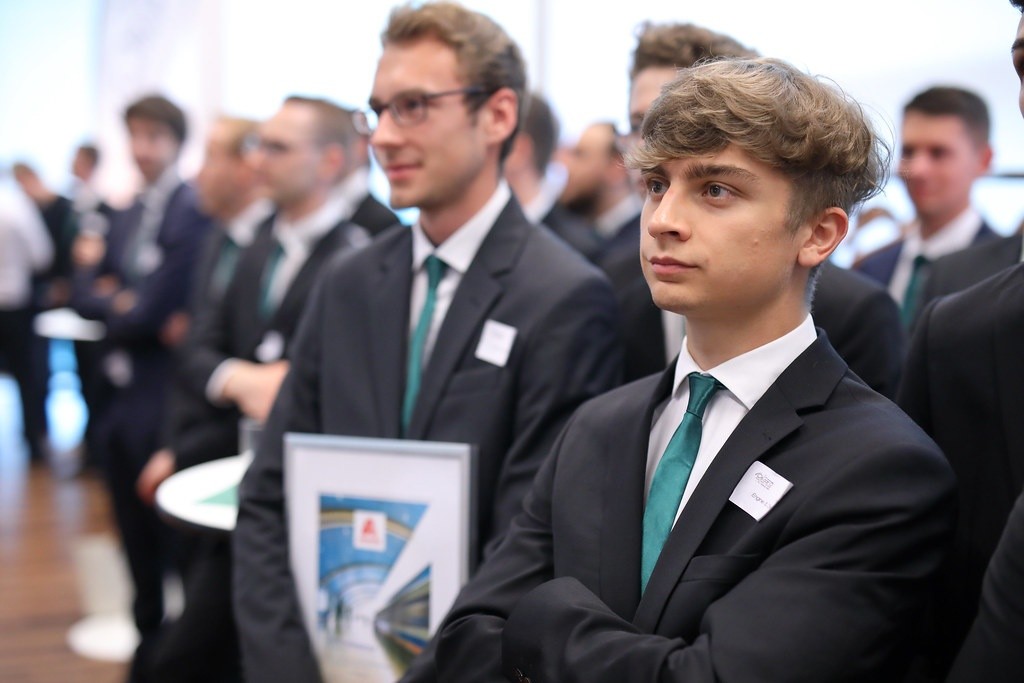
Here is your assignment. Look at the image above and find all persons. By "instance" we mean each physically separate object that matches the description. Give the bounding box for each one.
[230,1,625,683]
[0,175,54,464]
[603,20,903,403]
[68,96,211,407]
[112,98,402,682]
[502,94,606,261]
[886,0,1024,682]
[852,87,1003,305]
[13,165,72,300]
[397,58,956,683]
[568,118,641,235]
[69,146,120,468]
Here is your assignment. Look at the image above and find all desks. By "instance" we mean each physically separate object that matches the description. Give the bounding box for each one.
[156,449,257,531]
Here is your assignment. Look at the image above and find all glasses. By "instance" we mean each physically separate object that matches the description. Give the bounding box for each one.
[350,89,490,133]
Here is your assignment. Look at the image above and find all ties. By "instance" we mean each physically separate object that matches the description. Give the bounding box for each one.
[641,372,725,602]
[898,257,925,328]
[254,245,283,319]
[214,248,236,294]
[401,254,449,437]
